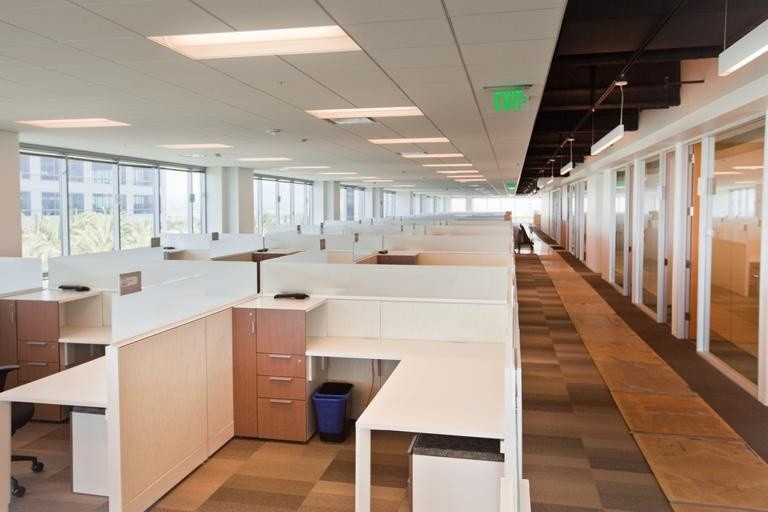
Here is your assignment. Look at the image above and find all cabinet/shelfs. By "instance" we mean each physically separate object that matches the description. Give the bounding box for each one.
[0,288,104,423]
[233,298,328,446]
[251,249,298,261]
[376,251,419,264]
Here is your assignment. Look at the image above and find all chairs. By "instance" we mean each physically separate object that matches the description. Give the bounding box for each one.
[1,364,45,499]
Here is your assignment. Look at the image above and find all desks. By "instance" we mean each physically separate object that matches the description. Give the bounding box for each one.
[305,332,504,512]
[0,324,160,512]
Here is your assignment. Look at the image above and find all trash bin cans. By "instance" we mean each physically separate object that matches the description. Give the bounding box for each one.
[310,382,354,443]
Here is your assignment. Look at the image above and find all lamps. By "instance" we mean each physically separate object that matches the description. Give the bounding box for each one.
[588,78,628,156]
[559,133,574,175]
[718,0,768,78]
